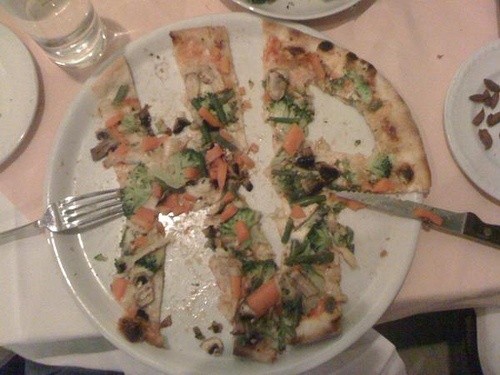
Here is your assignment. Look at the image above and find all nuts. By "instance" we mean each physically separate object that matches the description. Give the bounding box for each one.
[469,79,499,149]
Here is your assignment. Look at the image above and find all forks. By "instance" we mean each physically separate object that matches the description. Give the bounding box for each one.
[0,187,134,246]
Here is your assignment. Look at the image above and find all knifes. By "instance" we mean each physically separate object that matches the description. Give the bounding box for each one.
[333,189,500,248]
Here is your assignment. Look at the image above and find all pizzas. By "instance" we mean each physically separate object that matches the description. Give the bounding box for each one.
[93,18,431,364]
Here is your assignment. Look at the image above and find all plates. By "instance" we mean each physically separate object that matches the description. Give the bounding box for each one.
[42,11,426,375]
[216,0,362,22]
[443,34,499,210]
[0,25,41,175]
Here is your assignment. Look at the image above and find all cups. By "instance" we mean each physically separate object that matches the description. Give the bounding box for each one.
[0,1,107,70]
